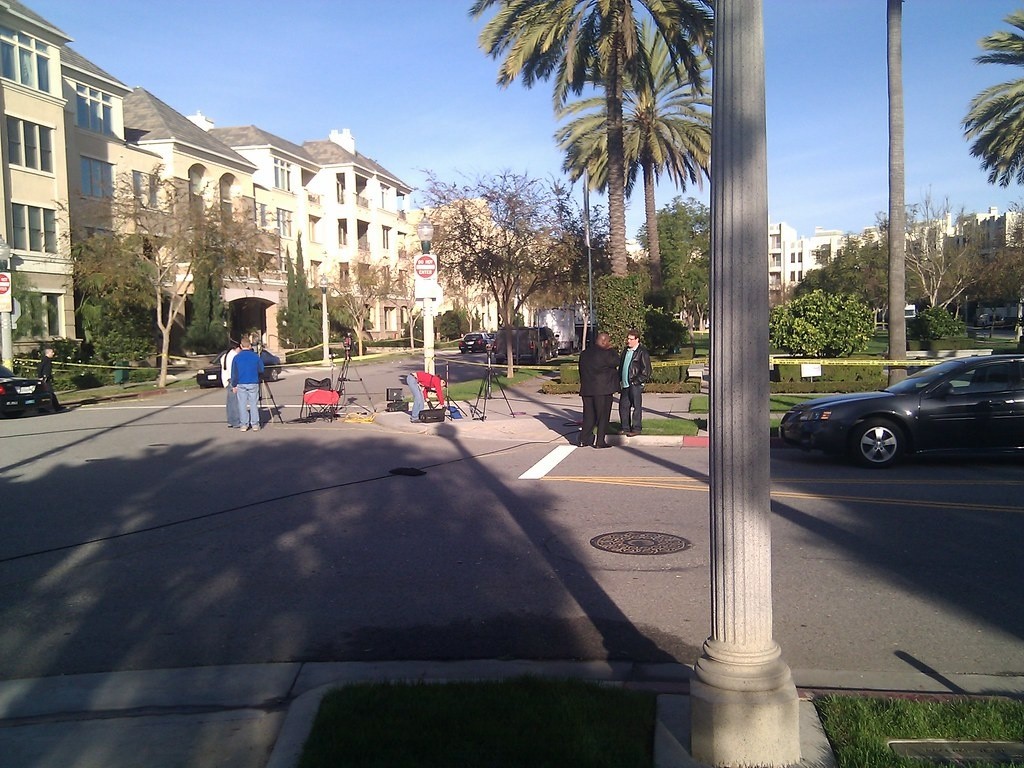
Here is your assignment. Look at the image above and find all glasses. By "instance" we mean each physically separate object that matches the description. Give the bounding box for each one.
[627,338,637,341]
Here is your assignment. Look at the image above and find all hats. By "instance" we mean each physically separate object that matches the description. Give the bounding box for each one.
[444,379,448,388]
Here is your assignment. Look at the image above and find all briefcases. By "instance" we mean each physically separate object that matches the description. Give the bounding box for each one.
[37,392,53,409]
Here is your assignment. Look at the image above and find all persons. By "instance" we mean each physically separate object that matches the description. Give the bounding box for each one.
[577,334,621,449]
[220,343,241,428]
[407,372,448,423]
[618,329,653,437]
[231,338,265,431]
[38,349,69,414]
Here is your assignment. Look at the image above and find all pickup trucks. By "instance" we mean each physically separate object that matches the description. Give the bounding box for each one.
[978,313,1005,327]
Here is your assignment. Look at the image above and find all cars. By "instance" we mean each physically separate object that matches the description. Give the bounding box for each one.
[492,326,559,365]
[0,364,52,419]
[459,333,494,352]
[196,346,281,388]
[778,356,1024,468]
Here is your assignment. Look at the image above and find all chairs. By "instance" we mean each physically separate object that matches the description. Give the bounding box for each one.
[968,368,986,390]
[987,365,1009,387]
[300,377,338,423]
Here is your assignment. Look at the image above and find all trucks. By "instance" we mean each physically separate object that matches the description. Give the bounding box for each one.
[536,308,580,354]
[879,302,915,323]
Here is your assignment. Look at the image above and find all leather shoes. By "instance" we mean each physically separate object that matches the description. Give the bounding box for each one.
[616,431,624,435]
[626,432,641,437]
[596,443,613,449]
[578,443,586,447]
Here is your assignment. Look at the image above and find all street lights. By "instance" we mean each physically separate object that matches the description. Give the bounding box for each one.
[319,278,329,359]
[0,235,12,356]
[416,215,435,373]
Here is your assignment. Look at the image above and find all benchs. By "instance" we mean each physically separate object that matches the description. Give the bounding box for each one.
[687,358,705,381]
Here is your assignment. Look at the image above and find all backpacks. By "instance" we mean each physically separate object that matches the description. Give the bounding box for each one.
[38,360,46,378]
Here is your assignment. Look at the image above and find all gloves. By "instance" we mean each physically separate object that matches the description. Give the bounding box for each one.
[427,401,434,410]
[442,407,446,411]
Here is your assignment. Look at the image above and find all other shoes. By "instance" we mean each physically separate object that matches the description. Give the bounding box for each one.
[253,425,262,432]
[42,410,49,414]
[410,419,423,423]
[240,427,248,432]
[55,406,66,413]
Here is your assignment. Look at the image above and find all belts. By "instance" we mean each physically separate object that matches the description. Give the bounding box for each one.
[410,373,417,379]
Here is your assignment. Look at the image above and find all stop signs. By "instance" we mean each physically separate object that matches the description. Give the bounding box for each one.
[0,274,9,295]
[416,256,436,278]
